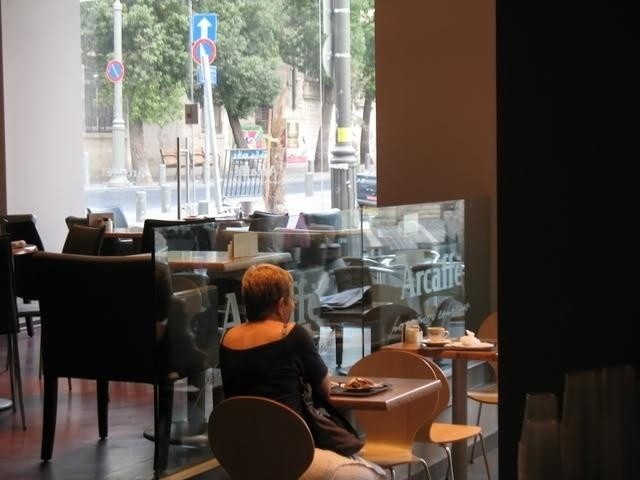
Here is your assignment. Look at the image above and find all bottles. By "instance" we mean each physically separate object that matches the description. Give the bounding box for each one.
[103,217,112,231]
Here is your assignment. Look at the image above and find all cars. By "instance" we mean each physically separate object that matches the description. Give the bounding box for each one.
[356,164,377,206]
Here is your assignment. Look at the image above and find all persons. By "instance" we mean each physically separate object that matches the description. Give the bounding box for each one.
[216,262,391,480]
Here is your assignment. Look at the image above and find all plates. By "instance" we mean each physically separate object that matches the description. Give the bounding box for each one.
[422,339,449,345]
[452,341,494,350]
[340,382,384,390]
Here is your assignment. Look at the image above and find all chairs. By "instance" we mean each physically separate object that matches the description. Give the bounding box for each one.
[1,208,499,480]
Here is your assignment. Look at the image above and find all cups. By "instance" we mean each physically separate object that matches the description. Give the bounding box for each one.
[426,326,449,342]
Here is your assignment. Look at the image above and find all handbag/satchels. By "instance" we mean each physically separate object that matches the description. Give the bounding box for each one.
[307,399,366,456]
